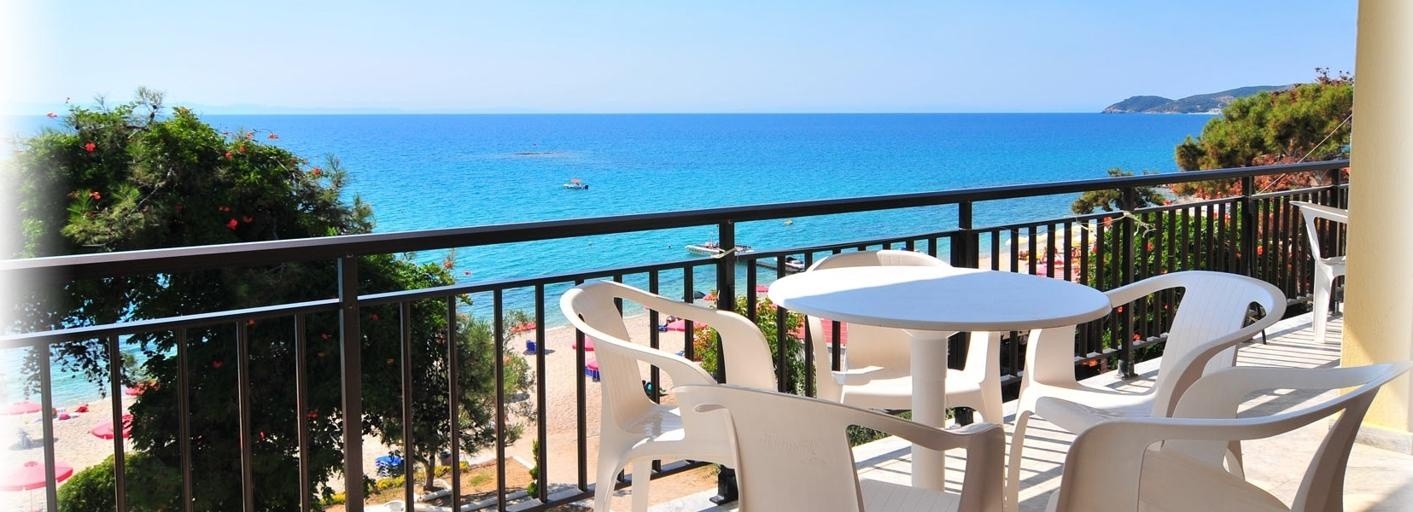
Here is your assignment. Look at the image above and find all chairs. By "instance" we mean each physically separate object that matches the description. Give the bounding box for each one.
[561,278,777,512]
[1287,197,1349,346]
[1009,270,1288,511]
[678,383,1006,511]
[801,249,1004,427]
[1049,362,1413,511]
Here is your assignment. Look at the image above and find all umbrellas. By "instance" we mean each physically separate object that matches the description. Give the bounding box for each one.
[91,421,130,440]
[665,319,707,332]
[571,340,594,353]
[0,460,73,512]
[0,400,42,416]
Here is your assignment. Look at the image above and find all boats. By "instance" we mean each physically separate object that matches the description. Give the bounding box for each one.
[684,240,804,274]
[562,183,589,190]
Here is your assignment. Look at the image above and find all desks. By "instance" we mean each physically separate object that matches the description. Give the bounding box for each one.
[768,266,1113,492]
